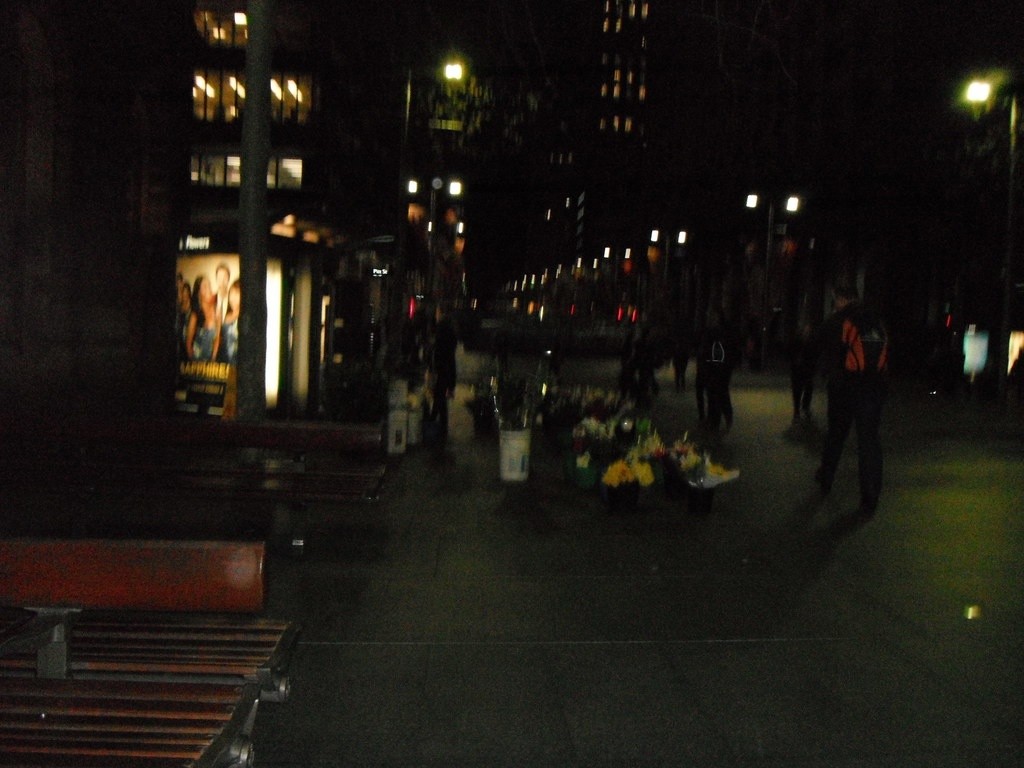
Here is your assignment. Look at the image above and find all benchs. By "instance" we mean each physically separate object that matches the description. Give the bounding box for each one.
[0,411,386,458]
[0,539,267,607]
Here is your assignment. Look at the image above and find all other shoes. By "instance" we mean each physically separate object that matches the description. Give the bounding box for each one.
[816,469,831,495]
[858,499,879,518]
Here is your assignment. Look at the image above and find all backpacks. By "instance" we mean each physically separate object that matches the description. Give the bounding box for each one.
[841,311,888,374]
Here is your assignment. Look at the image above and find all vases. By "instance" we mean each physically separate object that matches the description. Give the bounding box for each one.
[606,482,631,512]
[500,432,531,481]
[683,478,714,514]
[472,401,492,435]
[662,454,685,493]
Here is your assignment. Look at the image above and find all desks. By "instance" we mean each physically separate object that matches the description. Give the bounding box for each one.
[0,602,304,768]
[0,437,384,519]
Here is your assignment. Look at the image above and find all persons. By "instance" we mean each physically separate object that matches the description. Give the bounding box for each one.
[786,319,821,423]
[621,338,663,410]
[696,328,717,430]
[177,262,241,363]
[427,302,457,457]
[716,330,741,430]
[813,282,893,515]
[673,336,689,393]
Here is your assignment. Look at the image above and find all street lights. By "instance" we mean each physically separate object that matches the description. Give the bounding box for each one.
[957,70,1018,409]
[422,56,466,343]
[507,229,687,320]
[747,191,802,374]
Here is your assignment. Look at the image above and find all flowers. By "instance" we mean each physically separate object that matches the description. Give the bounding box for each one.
[468,350,741,488]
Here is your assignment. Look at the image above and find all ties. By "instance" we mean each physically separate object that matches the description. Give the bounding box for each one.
[219,301,223,320]
